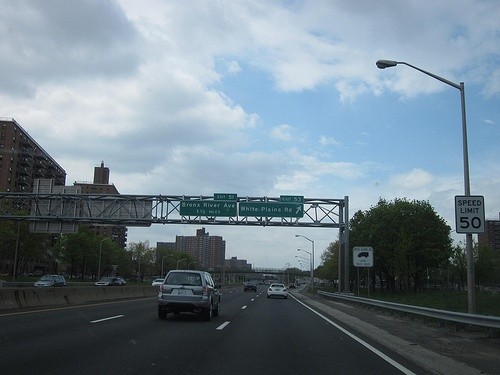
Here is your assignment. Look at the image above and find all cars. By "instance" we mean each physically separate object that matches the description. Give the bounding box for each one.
[267,284,289,299]
[33,275,66,288]
[289,281,306,290]
[244,282,257,292]
[151,278,165,287]
[93,276,126,286]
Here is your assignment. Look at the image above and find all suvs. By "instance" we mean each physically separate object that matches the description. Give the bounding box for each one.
[157,269,223,319]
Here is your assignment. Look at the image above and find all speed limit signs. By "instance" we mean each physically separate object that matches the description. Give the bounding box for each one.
[454,194,486,234]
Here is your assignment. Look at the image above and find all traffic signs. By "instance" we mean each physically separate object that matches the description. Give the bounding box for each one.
[239,202,304,218]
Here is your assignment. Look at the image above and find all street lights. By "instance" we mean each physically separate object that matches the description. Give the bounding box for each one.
[188,261,197,269]
[177,258,187,269]
[297,248,312,280]
[295,235,315,289]
[161,254,174,278]
[98,235,118,281]
[375,58,475,314]
